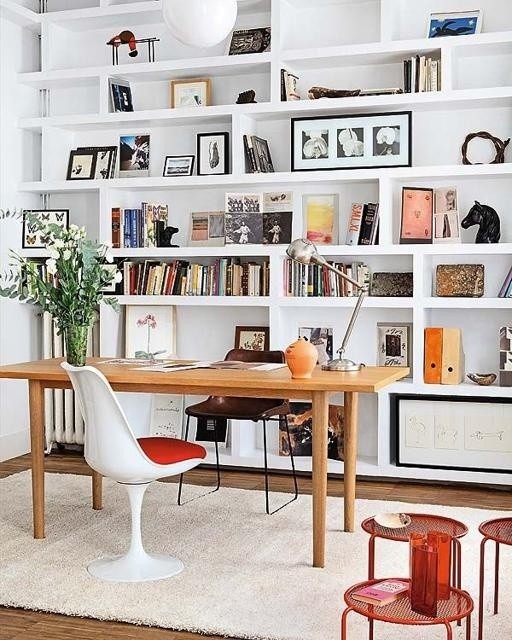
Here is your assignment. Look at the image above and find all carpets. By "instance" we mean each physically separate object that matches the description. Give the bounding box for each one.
[0,468,512,639]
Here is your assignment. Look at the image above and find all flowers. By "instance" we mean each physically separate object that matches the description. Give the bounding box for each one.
[0,207,127,362]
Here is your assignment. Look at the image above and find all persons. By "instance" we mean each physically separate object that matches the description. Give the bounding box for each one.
[268,221,283,243]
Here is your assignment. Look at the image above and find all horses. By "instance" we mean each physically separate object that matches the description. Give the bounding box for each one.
[461,200,500,243]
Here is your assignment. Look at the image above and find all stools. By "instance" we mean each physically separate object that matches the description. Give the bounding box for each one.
[342,577,474,640]
[478,517,512,639]
[362,513,469,626]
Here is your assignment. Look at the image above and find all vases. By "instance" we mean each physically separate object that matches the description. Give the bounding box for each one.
[64,326,88,367]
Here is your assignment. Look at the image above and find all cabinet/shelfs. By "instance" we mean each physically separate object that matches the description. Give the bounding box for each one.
[9,0,512,487]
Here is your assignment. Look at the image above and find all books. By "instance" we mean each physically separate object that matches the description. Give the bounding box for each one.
[351,578,410,608]
[498,267,511,298]
[284,72,303,102]
[505,282,512,298]
[402,55,441,94]
[377,326,409,367]
[371,205,380,246]
[109,190,271,297]
[368,203,378,245]
[109,78,135,113]
[298,327,335,363]
[67,130,153,181]
[359,203,377,246]
[281,258,370,298]
[280,69,299,102]
[358,87,404,97]
[344,202,365,246]
[261,191,293,245]
[20,260,64,299]
[243,133,274,174]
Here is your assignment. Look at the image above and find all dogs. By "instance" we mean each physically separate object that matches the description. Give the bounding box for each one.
[161,226,180,247]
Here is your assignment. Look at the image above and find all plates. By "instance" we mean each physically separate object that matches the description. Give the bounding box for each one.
[373,511,412,531]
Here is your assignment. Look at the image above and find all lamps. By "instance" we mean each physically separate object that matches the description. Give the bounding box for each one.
[286,238,366,371]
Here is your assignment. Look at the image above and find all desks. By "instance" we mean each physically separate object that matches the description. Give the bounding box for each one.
[0,357,411,567]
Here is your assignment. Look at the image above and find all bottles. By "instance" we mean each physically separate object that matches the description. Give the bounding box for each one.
[285,337,319,380]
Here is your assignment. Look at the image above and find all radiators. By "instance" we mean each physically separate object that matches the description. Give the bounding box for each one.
[38,310,99,455]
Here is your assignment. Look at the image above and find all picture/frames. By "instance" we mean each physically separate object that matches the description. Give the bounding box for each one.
[77,147,117,179]
[163,155,194,176]
[235,325,268,351]
[291,111,412,171]
[66,150,95,179]
[171,79,210,108]
[196,132,229,174]
[125,305,176,359]
[23,209,69,248]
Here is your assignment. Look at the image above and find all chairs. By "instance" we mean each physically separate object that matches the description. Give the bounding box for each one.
[60,361,205,583]
[178,348,298,515]
